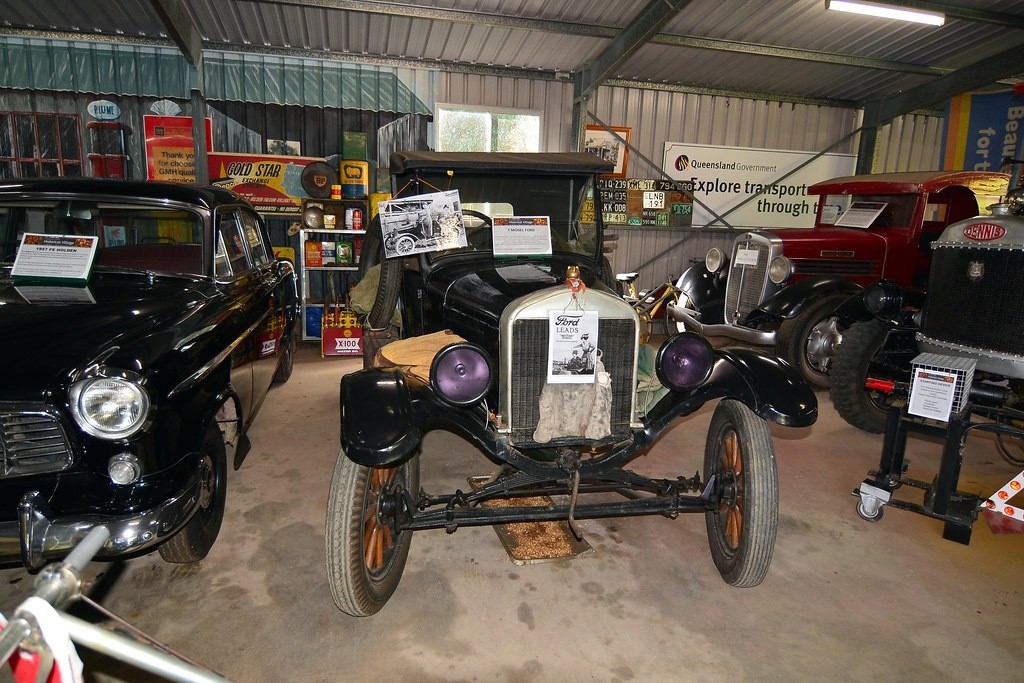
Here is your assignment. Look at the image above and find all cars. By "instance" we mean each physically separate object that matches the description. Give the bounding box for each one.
[1,177,298,570]
[828,182,1024,546]
[324,151,821,618]
[674,166,1024,435]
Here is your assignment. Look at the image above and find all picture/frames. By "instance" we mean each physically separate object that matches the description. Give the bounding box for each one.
[585,124,632,179]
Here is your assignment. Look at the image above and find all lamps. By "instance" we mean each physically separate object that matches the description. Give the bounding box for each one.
[825,0,948,28]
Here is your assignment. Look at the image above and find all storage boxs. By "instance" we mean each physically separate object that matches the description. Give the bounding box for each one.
[335,242,353,264]
[321,242,335,265]
[305,240,322,267]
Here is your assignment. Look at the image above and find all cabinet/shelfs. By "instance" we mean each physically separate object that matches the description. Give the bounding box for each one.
[299,229,366,348]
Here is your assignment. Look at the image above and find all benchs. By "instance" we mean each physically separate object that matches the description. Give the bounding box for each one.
[94,242,202,275]
